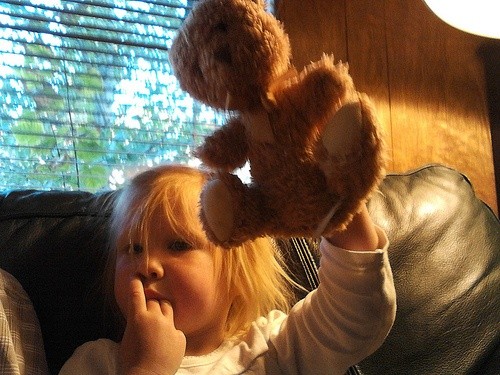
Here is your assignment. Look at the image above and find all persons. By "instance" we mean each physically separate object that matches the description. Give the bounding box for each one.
[60,166,396,375]
[0,270,46,375]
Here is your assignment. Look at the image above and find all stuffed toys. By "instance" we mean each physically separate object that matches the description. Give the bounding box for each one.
[169,1,387,249]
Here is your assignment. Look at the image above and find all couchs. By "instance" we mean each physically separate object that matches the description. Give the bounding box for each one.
[0,163,500,375]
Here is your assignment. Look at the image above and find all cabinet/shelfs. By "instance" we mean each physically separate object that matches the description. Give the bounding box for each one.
[269,0,500,228]
